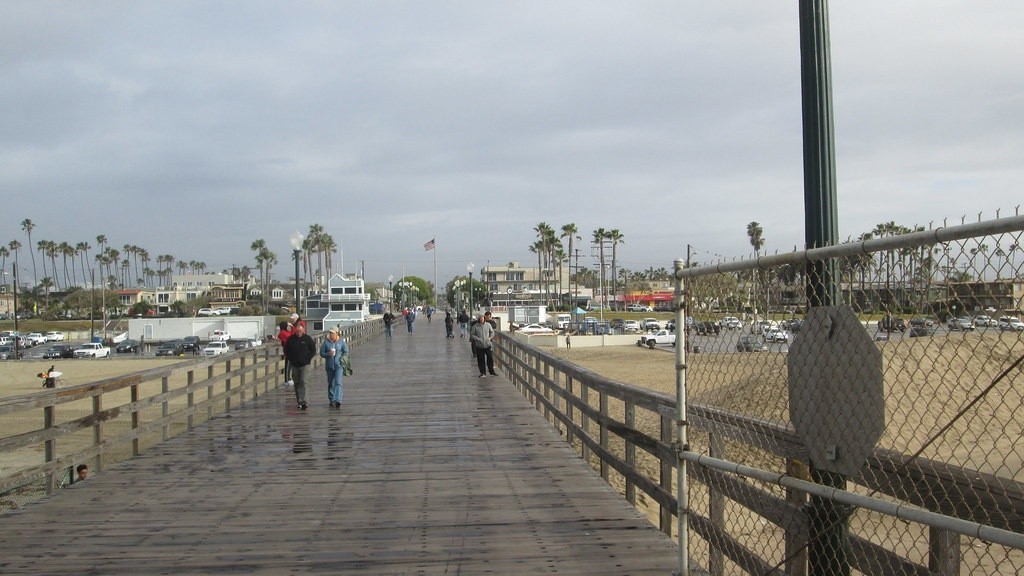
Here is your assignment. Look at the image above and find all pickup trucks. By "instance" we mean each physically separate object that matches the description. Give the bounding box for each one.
[43,344,79,358]
[73,342,111,360]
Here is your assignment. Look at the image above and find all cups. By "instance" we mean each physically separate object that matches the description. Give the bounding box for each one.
[331,349,335,355]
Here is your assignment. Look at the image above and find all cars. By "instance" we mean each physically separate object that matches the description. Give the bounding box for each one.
[156,330,249,357]
[508,312,1024,354]
[0,307,242,320]
[0,330,65,361]
[116,339,140,352]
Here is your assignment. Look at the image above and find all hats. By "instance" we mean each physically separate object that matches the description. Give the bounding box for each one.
[289,313,299,319]
[329,326,339,333]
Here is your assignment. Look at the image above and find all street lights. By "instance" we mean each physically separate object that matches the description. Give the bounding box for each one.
[288,230,304,322]
[466,262,476,358]
[388,275,394,315]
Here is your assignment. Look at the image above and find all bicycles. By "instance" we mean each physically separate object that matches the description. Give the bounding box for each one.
[42,377,67,389]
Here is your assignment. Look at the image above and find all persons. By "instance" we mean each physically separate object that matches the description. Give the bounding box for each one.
[469,316,478,359]
[278,313,306,385]
[285,324,316,408]
[406,310,415,334]
[485,311,497,330]
[193,341,197,355]
[423,305,432,322]
[402,304,417,320]
[320,326,349,407]
[43,365,55,388]
[383,311,395,338]
[566,335,570,349]
[459,310,468,338]
[470,315,499,377]
[445,313,454,338]
[74,464,88,483]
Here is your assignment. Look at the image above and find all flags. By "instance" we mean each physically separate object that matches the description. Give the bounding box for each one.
[424,239,435,251]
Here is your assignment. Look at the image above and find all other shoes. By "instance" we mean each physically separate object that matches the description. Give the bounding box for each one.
[460,334,463,337]
[478,373,486,378]
[285,380,294,385]
[297,404,302,408]
[302,401,308,409]
[329,401,335,406]
[490,372,499,376]
[335,401,341,407]
